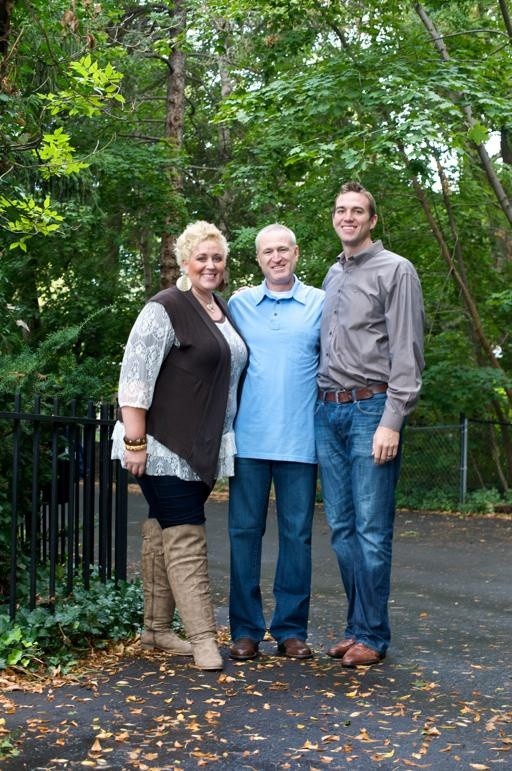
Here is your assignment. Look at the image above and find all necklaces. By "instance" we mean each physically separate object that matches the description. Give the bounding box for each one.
[192,292,218,312]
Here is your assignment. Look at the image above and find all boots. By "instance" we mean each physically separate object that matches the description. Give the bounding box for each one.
[139,518,193,657]
[161,523,224,671]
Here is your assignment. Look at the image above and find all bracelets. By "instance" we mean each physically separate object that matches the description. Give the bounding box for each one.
[123,435,147,445]
[124,444,147,451]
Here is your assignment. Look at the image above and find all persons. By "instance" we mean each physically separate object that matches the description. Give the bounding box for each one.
[321,181,425,666]
[227,223,327,661]
[110,219,254,671]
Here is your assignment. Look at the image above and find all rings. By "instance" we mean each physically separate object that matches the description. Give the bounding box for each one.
[388,455,393,458]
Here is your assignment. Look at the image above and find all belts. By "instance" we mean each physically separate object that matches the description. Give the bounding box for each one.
[318,384,386,402]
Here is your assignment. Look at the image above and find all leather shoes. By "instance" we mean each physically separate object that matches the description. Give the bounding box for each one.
[329,636,356,657]
[279,639,313,659]
[341,643,387,667]
[231,637,260,659]
